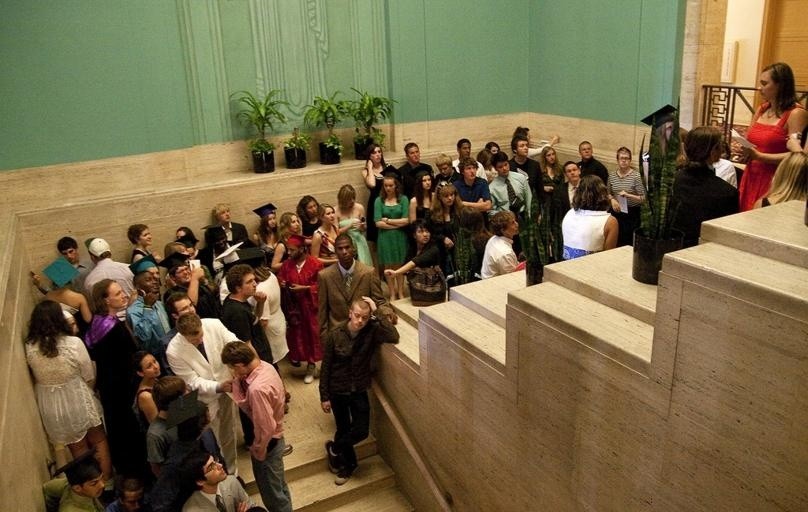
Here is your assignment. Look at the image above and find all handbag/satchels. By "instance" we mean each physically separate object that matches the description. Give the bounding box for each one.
[406,264,446,306]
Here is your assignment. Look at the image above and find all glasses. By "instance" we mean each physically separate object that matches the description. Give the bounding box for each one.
[178,302,195,313]
[617,157,632,160]
[566,169,579,173]
[205,455,221,475]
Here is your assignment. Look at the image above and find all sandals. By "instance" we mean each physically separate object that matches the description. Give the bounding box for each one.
[291,359,301,367]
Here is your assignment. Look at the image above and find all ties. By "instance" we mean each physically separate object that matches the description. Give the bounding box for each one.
[505,178,517,206]
[214,494,226,512]
[344,272,353,300]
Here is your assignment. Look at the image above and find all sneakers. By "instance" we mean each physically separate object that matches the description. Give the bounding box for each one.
[303,363,317,383]
[324,439,338,472]
[285,406,289,413]
[281,445,294,454]
[335,464,361,484]
[282,393,290,402]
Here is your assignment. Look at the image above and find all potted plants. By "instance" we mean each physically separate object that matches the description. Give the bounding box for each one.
[445,227,485,302]
[632,95,686,284]
[299,90,348,165]
[509,185,562,288]
[227,87,291,174]
[342,84,399,161]
[280,122,311,170]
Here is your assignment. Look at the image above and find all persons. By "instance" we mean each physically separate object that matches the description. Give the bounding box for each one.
[451,139,487,181]
[489,151,532,223]
[508,136,547,212]
[252,203,278,252]
[176,227,200,250]
[220,264,293,457]
[23,300,109,479]
[195,224,238,278]
[426,180,463,275]
[432,153,460,189]
[452,158,492,212]
[767,107,778,118]
[485,142,499,155]
[275,234,325,384]
[640,105,680,190]
[157,251,223,318]
[296,195,322,236]
[271,212,308,270]
[236,247,291,414]
[221,341,293,512]
[574,141,608,186]
[733,63,808,211]
[165,313,247,489]
[105,475,150,512]
[311,203,340,265]
[39,255,92,329]
[164,243,190,256]
[397,142,433,198]
[173,234,216,279]
[132,350,161,426]
[245,506,268,512]
[538,146,564,226]
[56,302,79,335]
[607,148,646,248]
[670,128,737,188]
[128,253,161,283]
[374,173,409,303]
[42,449,105,512]
[30,237,92,294]
[319,296,400,485]
[561,161,582,216]
[476,151,498,182]
[212,203,248,248]
[85,279,142,472]
[160,292,195,375]
[786,124,808,155]
[127,224,162,296]
[362,144,388,237]
[480,211,519,280]
[126,270,172,369]
[561,175,619,260]
[150,388,228,511]
[181,453,256,512]
[668,126,739,247]
[213,241,243,305]
[81,237,135,320]
[753,152,808,210]
[333,185,373,268]
[146,376,192,476]
[317,233,398,354]
[510,127,559,160]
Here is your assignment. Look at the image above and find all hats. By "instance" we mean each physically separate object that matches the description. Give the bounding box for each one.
[235,246,266,267]
[285,230,313,250]
[61,303,80,318]
[165,387,208,440]
[128,254,159,273]
[640,104,678,129]
[83,237,111,256]
[215,239,244,264]
[380,165,401,177]
[156,251,190,269]
[41,255,80,287]
[52,447,102,487]
[252,203,278,217]
[176,233,199,249]
[201,220,226,240]
